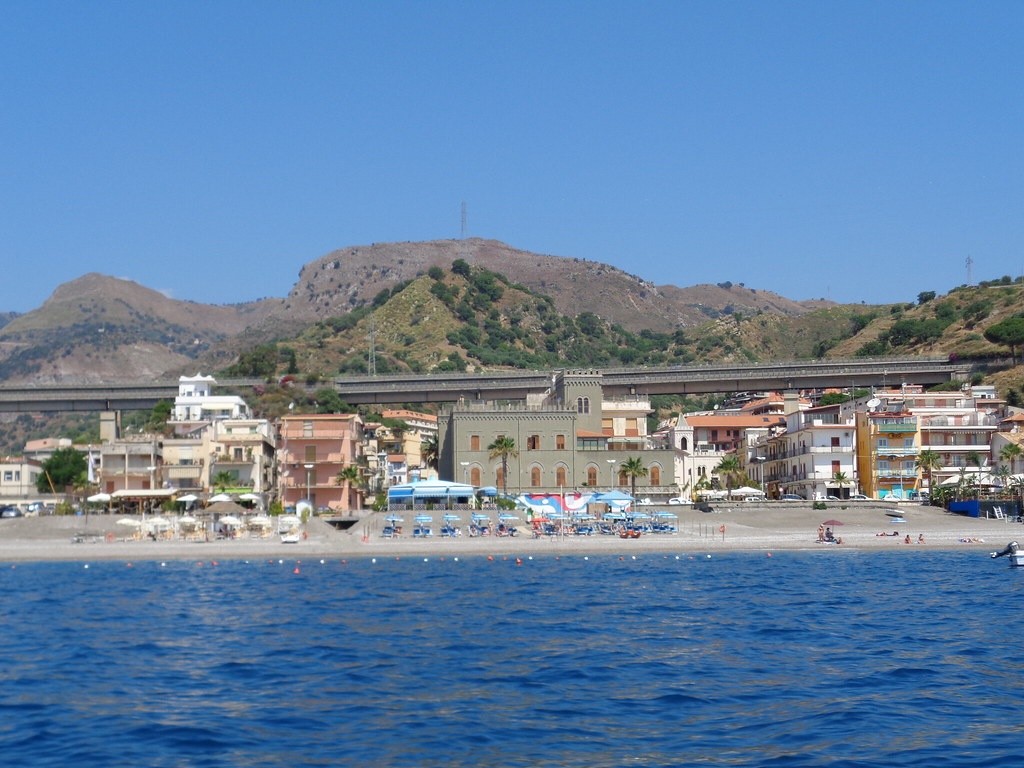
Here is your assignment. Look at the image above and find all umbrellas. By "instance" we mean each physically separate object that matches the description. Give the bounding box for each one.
[384,511,520,538]
[239,493,259,499]
[207,494,233,501]
[250,517,268,533]
[651,510,678,529]
[218,516,240,539]
[596,489,635,513]
[823,520,843,532]
[117,518,165,526]
[478,486,497,496]
[178,508,217,522]
[548,513,649,526]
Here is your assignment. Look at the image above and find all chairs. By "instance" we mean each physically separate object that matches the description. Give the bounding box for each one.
[382,526,402,538]
[470,523,518,535]
[544,523,677,534]
[441,526,460,535]
[412,525,435,537]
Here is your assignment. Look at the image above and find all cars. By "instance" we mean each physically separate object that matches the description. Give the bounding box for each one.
[636,499,653,505]
[668,498,693,504]
[851,494,871,499]
[745,497,761,501]
[881,494,908,501]
[818,496,839,500]
[780,493,806,500]
[1,507,21,518]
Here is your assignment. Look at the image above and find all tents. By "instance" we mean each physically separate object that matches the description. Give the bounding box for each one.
[695,486,765,501]
[388,480,475,509]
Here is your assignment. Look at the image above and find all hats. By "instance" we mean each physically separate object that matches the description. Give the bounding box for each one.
[826,528,830,529]
[820,524,824,526]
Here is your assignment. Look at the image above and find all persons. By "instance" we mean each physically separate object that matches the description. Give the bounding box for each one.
[876,531,899,536]
[905,534,925,544]
[817,525,841,544]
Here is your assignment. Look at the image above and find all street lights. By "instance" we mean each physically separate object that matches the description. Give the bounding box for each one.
[688,455,696,493]
[607,460,616,490]
[755,456,766,501]
[304,464,313,499]
[460,462,469,484]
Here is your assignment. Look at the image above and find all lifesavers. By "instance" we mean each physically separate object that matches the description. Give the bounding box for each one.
[719,525,726,533]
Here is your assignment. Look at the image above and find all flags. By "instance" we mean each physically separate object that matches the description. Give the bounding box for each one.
[87,451,95,482]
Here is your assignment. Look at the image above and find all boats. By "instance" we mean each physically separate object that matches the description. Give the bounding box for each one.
[991,541,1024,566]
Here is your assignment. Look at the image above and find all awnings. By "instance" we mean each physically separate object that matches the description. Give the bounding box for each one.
[87,493,111,502]
[177,494,198,501]
